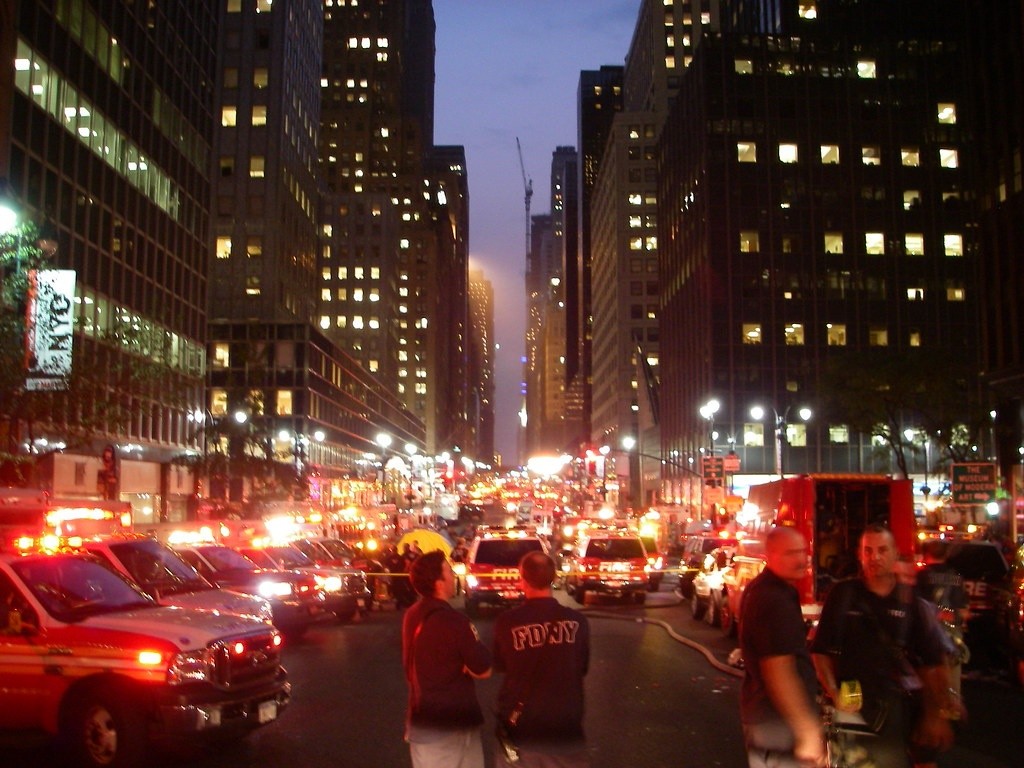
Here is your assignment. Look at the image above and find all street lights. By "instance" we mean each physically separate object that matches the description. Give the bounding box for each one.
[600,446,704,522]
[405,441,417,511]
[376,432,392,503]
[752,399,814,478]
[699,400,720,527]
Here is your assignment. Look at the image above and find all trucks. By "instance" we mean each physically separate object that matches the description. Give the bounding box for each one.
[719,474,917,655]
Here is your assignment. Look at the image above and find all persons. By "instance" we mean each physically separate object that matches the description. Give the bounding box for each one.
[350,523,592,768]
[808,524,954,768]
[736,525,825,768]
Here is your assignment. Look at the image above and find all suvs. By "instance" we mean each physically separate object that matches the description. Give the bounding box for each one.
[0,554,291,767]
[24,532,375,639]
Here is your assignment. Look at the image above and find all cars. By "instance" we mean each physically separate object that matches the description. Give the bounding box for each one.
[674,535,738,626]
[463,525,548,620]
[565,529,668,608]
